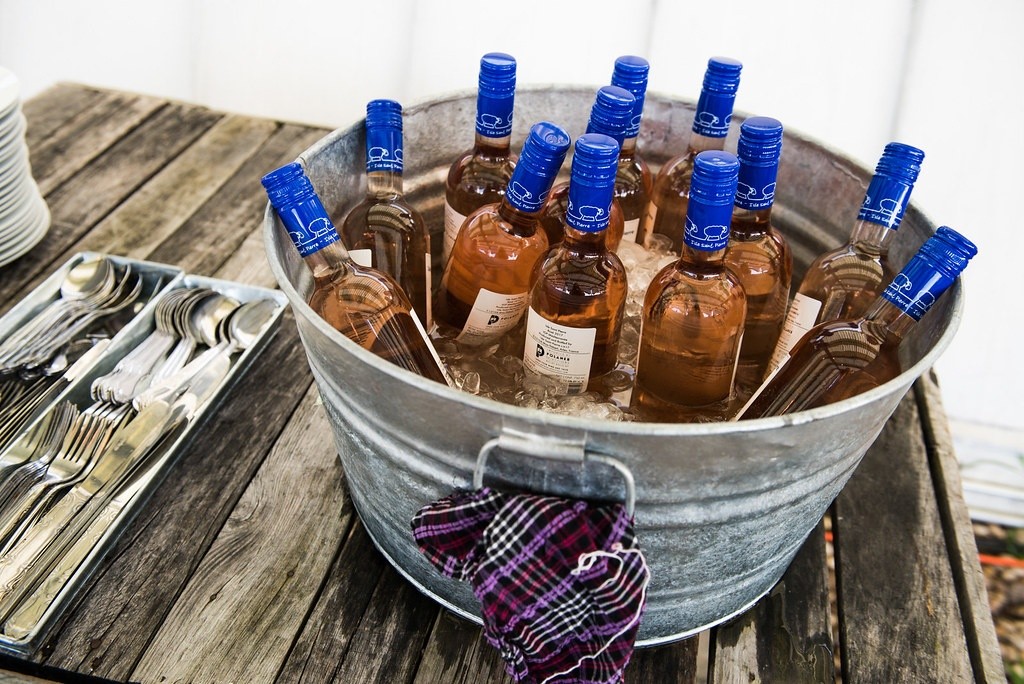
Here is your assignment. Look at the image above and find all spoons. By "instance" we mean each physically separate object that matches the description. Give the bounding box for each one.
[0,258,280,412]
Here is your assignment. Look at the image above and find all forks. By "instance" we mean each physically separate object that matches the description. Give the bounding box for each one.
[0,375,134,559]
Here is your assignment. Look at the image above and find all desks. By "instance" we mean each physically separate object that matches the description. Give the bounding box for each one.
[0,81,1009,684]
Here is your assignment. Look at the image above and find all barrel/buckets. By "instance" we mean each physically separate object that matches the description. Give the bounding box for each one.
[261,80,964,647]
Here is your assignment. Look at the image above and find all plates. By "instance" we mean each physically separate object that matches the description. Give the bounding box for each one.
[0,64,53,269]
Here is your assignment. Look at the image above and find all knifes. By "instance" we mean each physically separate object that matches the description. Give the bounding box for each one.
[0,393,197,639]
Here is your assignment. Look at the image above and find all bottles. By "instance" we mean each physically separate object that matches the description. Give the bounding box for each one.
[535,85,635,255]
[340,98,435,342]
[433,120,571,352]
[723,117,793,401]
[760,139,926,384]
[613,54,655,245]
[439,52,517,273]
[734,224,978,418]
[518,132,629,399]
[634,54,744,258]
[260,159,460,392]
[627,150,749,425]
